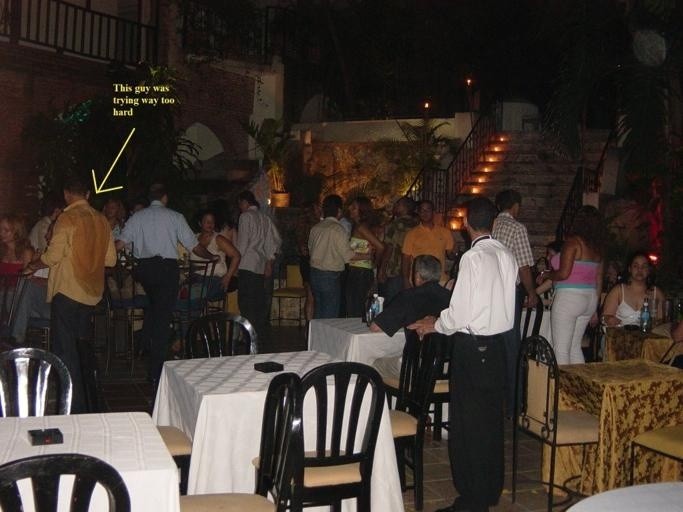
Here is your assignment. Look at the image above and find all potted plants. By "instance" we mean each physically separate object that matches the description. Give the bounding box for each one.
[244,117,297,208]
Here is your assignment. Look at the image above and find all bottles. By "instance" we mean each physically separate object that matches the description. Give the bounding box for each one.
[639,296,652,333]
[365,293,384,326]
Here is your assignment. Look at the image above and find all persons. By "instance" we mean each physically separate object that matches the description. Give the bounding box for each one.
[415,197,518,511]
[307,194,460,320]
[14,170,117,413]
[370,255,452,394]
[106,183,283,379]
[0,198,65,347]
[494,190,665,366]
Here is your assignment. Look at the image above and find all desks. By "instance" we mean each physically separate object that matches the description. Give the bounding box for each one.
[514,300,551,351]
[542,355,682,500]
[147,346,408,511]
[599,322,683,367]
[561,479,682,511]
[303,314,452,437]
[0,409,181,511]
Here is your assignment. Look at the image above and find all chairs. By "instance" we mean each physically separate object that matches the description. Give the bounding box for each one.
[0,343,76,416]
[509,280,542,351]
[149,421,195,497]
[249,347,389,510]
[0,244,314,373]
[401,319,453,448]
[385,328,455,512]
[625,420,683,487]
[0,453,135,511]
[180,314,262,357]
[510,331,605,510]
[178,374,303,512]
